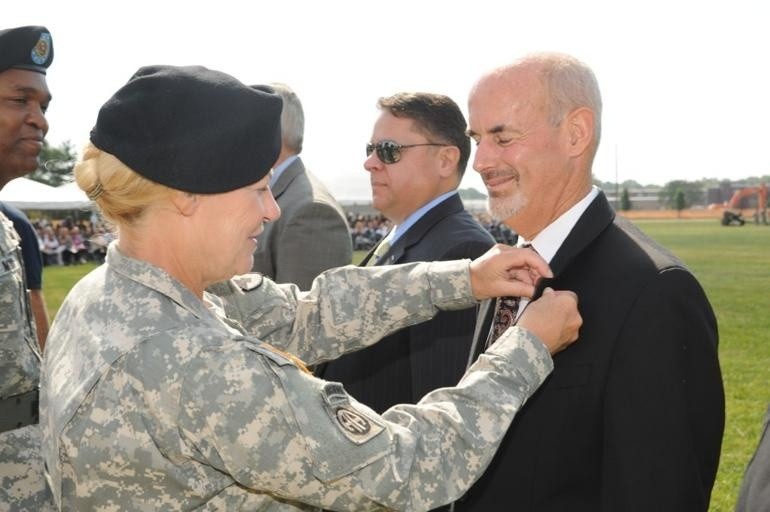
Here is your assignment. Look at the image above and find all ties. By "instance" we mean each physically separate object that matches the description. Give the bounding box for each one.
[489,242,534,346]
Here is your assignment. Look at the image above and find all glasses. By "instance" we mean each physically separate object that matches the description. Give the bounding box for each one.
[366,140,449,164]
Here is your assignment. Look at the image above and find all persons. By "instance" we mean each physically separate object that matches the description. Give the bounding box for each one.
[313,91,498,416]
[734,403,770,512]
[29,216,118,265]
[250,83,353,374]
[344,212,392,251]
[427,50,726,512]
[470,212,518,246]
[0,198,50,353]
[0,26,60,512]
[37,64,584,512]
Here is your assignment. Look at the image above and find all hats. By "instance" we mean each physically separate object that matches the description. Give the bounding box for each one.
[0,26,53,74]
[90,66,283,195]
